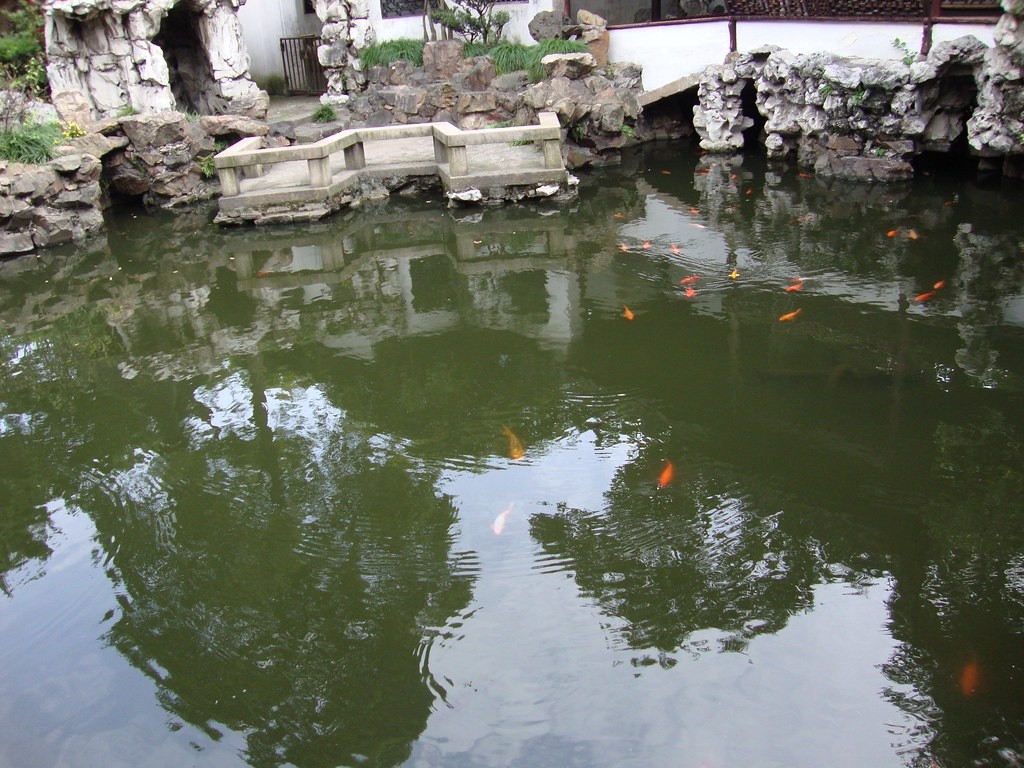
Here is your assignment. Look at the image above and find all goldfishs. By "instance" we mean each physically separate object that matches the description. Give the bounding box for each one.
[957,656,984,700]
[611,147,961,324]
[487,424,676,537]
